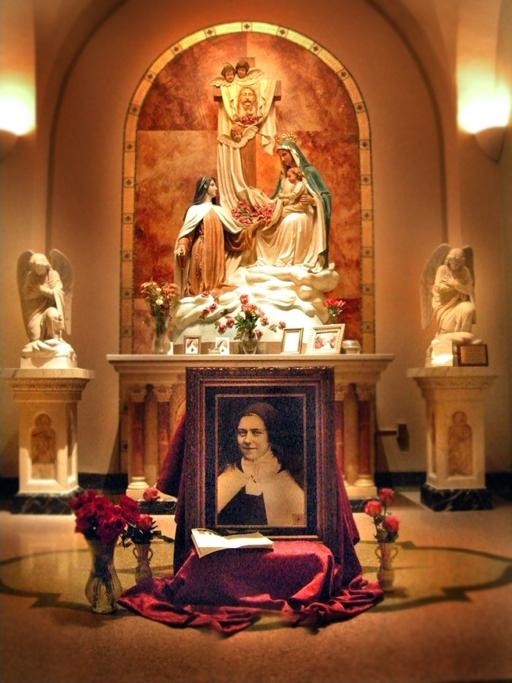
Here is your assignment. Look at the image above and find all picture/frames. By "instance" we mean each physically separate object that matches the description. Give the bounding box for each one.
[215,337,230,353]
[183,336,202,354]
[281,328,304,354]
[184,365,334,548]
[458,344,489,367]
[305,323,346,354]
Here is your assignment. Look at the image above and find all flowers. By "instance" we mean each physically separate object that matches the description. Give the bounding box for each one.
[364,487,400,543]
[321,296,346,323]
[69,487,125,607]
[199,292,286,341]
[140,280,180,337]
[118,488,160,544]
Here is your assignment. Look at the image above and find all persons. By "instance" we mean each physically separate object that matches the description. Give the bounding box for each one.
[22,253,65,341]
[235,61,250,79]
[231,125,244,143]
[216,402,305,527]
[176,176,260,298]
[432,248,476,332]
[237,87,258,115]
[222,64,235,82]
[278,167,309,220]
[255,139,332,273]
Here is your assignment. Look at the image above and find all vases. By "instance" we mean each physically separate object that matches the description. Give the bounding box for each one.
[133,543,154,585]
[242,338,257,354]
[150,316,170,353]
[375,543,399,593]
[85,537,124,613]
